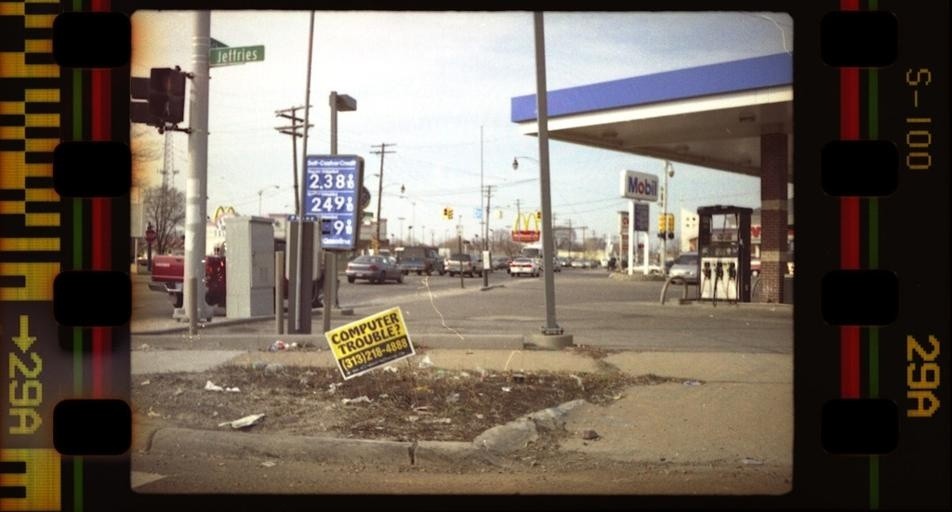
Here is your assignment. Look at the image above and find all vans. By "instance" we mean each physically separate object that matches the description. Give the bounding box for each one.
[397,245,444,276]
[520,246,561,272]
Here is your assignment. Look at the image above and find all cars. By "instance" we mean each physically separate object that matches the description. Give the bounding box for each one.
[345,253,405,285]
[444,253,484,277]
[668,249,698,285]
[559,255,598,269]
[508,257,540,277]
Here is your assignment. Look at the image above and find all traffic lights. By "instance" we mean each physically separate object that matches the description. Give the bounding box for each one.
[129,64,187,135]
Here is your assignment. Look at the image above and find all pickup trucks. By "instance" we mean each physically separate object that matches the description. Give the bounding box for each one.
[145,231,326,313]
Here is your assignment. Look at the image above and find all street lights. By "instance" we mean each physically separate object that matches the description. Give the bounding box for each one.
[662,161,675,276]
[323,91,358,307]
